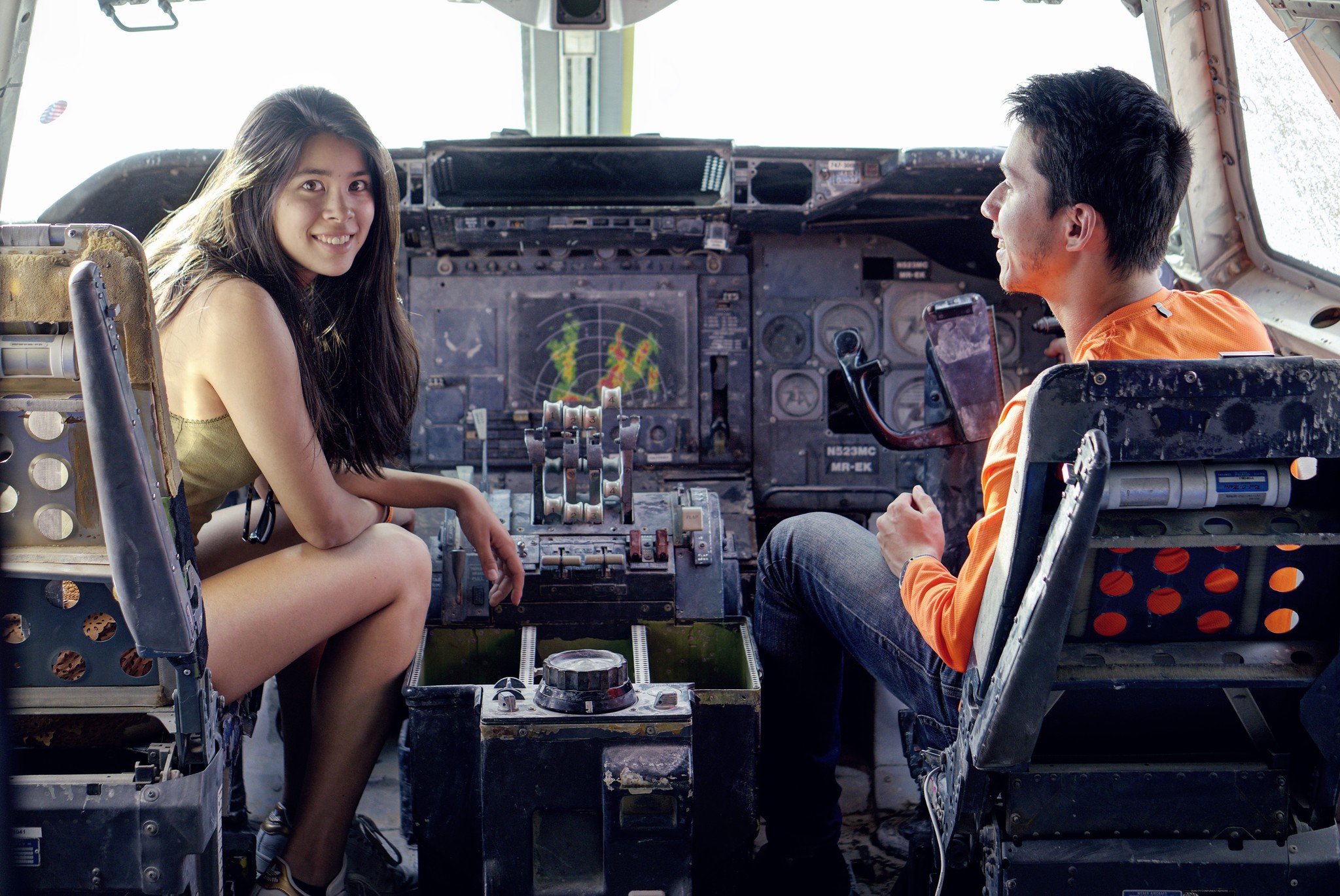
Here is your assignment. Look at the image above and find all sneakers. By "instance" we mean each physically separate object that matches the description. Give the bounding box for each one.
[251,851,387,895]
[256,800,419,894]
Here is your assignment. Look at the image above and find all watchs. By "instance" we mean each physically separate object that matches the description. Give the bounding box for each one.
[899,554,938,589]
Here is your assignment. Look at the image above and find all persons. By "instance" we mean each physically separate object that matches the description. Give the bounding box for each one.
[140,87,525,896]
[751,68,1277,896]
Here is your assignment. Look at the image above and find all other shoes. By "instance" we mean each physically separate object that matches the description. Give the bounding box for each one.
[690,839,856,896]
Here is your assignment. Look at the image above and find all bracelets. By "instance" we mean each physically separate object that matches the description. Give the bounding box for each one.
[385,506,393,523]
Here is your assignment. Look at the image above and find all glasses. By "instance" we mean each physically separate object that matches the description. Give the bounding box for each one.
[242,481,276,545]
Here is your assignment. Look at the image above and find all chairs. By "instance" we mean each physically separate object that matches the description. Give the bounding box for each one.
[0,222,267,895]
[896,355,1339,896]
